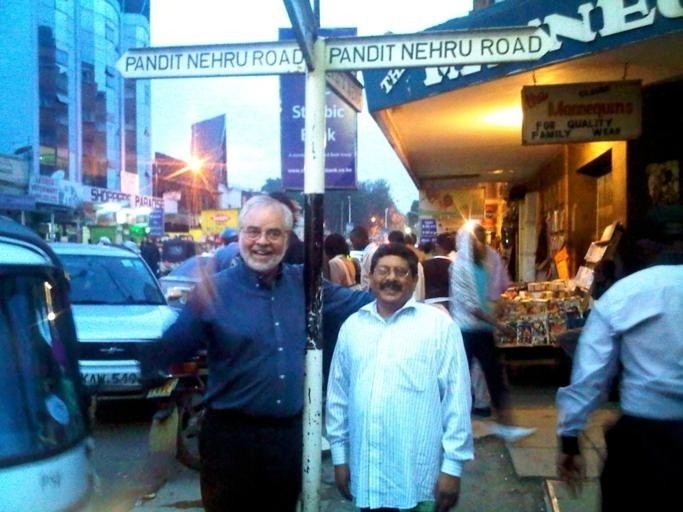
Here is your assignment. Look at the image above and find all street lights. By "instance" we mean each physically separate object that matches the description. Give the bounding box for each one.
[384,207,388,230]
[369,217,375,227]
[186,151,201,229]
[347,194,351,225]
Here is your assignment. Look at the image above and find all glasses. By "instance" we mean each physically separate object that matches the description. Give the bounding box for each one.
[369,265,414,281]
[236,224,287,241]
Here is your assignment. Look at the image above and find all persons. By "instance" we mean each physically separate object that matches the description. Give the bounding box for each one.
[140,193,517,511]
[554,204,683,512]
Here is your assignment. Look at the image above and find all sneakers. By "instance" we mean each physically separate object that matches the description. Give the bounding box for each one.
[495,423,535,443]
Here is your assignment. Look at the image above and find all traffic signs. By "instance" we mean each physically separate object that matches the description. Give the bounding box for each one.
[115,42,306,78]
[326,68,366,113]
[282,0,315,70]
[323,24,553,67]
